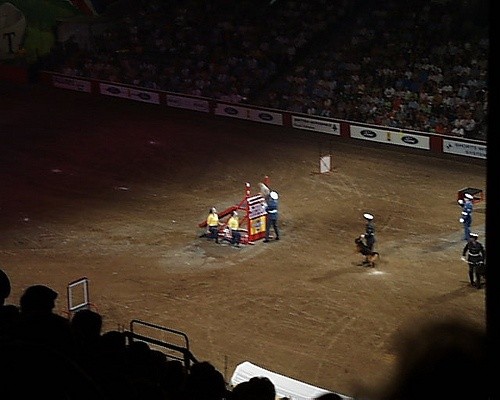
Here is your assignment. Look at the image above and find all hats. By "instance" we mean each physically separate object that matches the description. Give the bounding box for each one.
[470,232,478,239]
[464,193,474,200]
[270,191,279,200]
[363,212,374,221]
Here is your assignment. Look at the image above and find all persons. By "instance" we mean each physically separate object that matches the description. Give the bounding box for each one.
[63,1,488,140]
[258,189,279,242]
[463,233,485,288]
[207,207,224,244]
[228,211,241,249]
[458,193,473,240]
[0,270,276,400]
[361,214,376,266]
[281,318,490,400]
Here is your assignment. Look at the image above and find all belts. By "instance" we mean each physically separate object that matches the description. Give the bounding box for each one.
[461,212,472,215]
[468,252,479,256]
[268,210,277,213]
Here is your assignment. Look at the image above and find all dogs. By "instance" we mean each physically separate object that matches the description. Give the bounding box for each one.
[355,236,379,268]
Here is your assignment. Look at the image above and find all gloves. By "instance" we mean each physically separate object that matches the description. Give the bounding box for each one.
[459,218,464,223]
[461,257,466,261]
[360,234,364,238]
